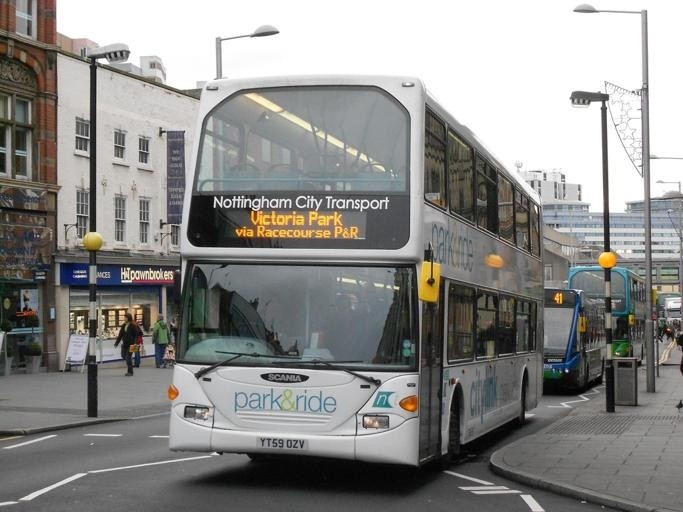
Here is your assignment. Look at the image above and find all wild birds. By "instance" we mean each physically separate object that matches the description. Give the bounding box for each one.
[676,400,683,410]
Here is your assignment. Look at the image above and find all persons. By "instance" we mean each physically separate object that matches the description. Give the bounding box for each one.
[169,318,177,345]
[114,313,138,376]
[132,320,143,368]
[22,295,31,311]
[151,315,170,368]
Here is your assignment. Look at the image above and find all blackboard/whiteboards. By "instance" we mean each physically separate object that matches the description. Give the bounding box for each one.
[64,335,89,364]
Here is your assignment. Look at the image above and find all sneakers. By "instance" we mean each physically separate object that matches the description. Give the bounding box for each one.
[125,372,133,376]
[133,364,139,368]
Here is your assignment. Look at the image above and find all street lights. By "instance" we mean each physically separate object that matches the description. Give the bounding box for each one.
[216,25,280,78]
[514,158,522,174]
[570,4,655,393]
[89,43,131,417]
[656,180,681,195]
[650,153,683,160]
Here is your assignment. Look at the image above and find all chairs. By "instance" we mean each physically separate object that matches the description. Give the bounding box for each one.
[223,162,406,193]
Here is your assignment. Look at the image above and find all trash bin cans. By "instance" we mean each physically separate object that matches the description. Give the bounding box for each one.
[613,358,638,406]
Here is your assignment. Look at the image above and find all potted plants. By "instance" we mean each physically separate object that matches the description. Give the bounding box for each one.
[20,341,45,374]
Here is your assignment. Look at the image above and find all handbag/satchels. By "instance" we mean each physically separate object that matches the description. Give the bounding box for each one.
[129,344,140,353]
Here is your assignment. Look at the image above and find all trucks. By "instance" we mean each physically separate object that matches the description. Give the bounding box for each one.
[656,292,681,318]
